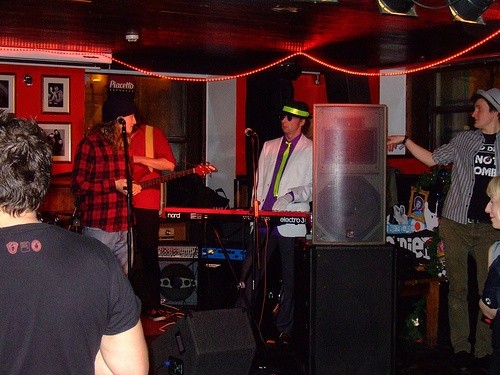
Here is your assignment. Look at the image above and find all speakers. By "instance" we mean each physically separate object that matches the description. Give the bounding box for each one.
[308,246,398,375]
[156,246,247,312]
[146,307,256,375]
[312,104,387,245]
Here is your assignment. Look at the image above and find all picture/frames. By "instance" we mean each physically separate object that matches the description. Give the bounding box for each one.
[407,187,430,222]
[36,121,72,164]
[0,73,16,117]
[40,75,71,115]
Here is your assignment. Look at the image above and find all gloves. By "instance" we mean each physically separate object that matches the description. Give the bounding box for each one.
[272,193,293,212]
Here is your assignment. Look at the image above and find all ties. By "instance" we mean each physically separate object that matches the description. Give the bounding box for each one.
[273,140,292,197]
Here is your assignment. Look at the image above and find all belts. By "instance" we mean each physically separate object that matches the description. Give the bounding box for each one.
[467,217,482,224]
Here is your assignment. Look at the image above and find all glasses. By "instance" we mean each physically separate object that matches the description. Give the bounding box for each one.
[279,114,297,121]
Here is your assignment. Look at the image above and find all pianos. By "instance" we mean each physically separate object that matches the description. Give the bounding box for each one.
[161,206,313,375]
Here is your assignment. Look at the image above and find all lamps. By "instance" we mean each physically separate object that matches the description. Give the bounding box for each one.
[126,26,139,42]
[446,0,494,26]
[377,0,418,17]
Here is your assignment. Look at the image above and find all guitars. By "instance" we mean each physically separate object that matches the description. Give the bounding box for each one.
[131,161,220,190]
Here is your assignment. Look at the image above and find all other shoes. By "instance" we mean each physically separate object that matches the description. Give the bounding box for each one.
[280,332,292,344]
[456,351,493,375]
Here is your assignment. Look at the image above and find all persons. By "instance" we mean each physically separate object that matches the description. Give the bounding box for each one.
[72,81,177,316]
[0,107,150,375]
[250,101,317,348]
[478,176,500,375]
[385,88,500,375]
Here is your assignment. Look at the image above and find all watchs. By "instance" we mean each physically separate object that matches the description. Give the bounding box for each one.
[402,135,410,145]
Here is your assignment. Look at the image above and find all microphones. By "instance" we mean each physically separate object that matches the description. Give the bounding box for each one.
[117,117,126,125]
[245,128,257,136]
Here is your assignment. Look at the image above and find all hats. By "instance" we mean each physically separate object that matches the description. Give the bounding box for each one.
[477,88,500,112]
[102,95,138,123]
[281,101,313,119]
